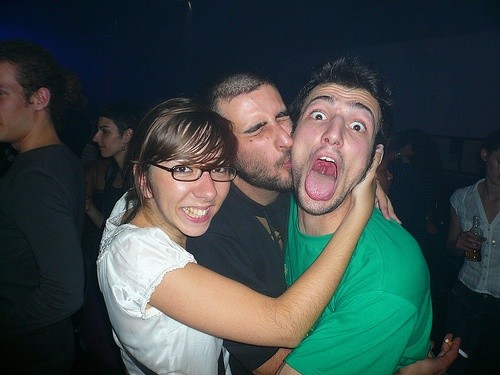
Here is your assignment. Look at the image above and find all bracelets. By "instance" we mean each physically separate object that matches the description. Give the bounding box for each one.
[392,149,401,160]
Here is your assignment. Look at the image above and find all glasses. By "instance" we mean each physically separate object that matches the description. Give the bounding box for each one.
[150,163,237,183]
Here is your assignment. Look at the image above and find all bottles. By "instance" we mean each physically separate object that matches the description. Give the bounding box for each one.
[466,215,484,261]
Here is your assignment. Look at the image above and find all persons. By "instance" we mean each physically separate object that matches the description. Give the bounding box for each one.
[0,37,83,375]
[98,97,384,374]
[275,57,432,375]
[438,130,500,375]
[185,74,461,375]
[377,129,444,244]
[84,109,135,375]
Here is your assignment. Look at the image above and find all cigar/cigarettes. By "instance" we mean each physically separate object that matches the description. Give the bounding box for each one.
[443,339,468,358]
